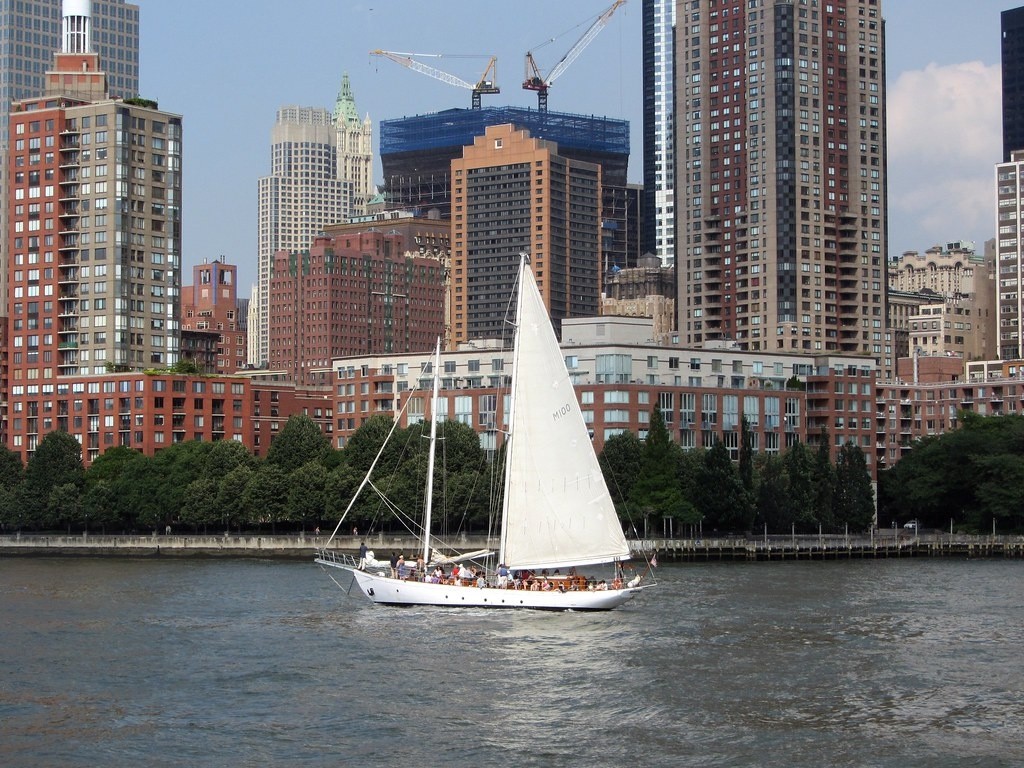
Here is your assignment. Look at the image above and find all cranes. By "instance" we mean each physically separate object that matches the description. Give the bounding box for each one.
[522,0,631,139]
[367,49,500,109]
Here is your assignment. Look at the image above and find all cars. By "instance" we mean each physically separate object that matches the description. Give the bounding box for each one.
[905,520,922,528]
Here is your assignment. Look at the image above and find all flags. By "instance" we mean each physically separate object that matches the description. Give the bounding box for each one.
[650,555,657,567]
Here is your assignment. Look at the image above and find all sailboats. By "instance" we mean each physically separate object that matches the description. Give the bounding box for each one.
[312,250,662,611]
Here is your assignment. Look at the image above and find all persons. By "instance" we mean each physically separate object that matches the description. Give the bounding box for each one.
[390,551,627,593]
[353,527,357,535]
[358,542,368,570]
[316,527,320,534]
